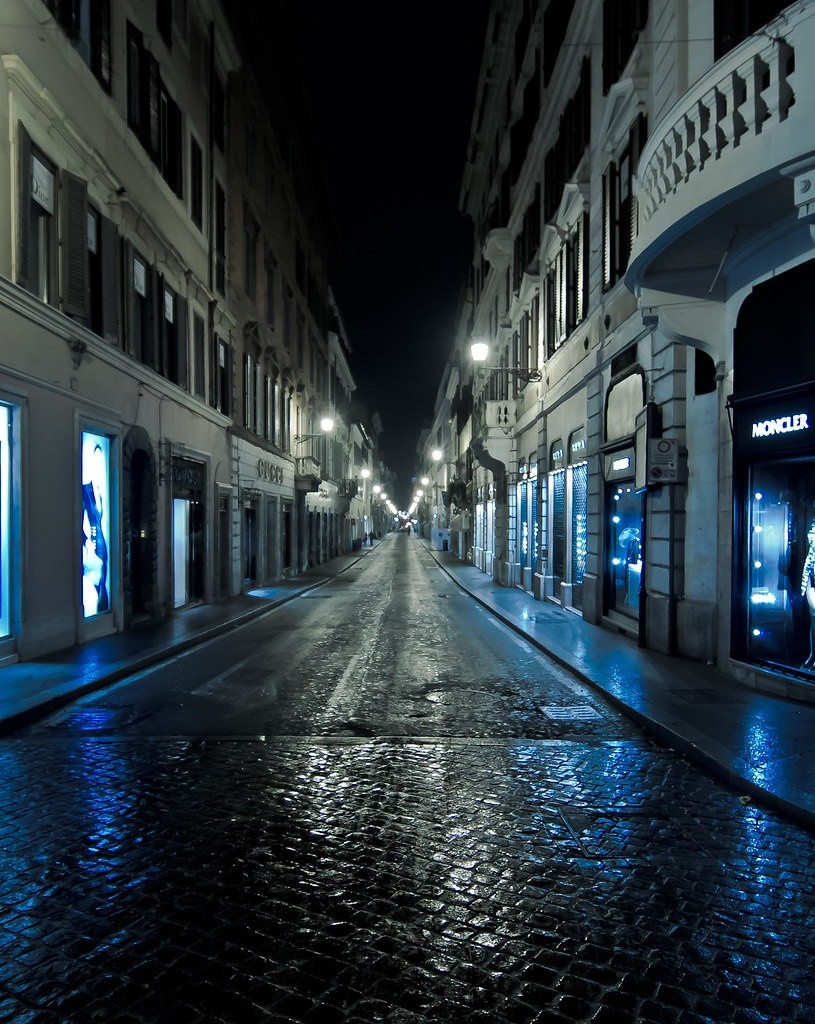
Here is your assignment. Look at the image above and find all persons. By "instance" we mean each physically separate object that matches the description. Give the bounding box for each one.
[363,530,374,546]
[83,444,108,618]
[799,529,815,671]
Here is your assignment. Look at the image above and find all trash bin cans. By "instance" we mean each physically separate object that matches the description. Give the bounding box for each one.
[353,538,361,551]
[443,539,448,551]
